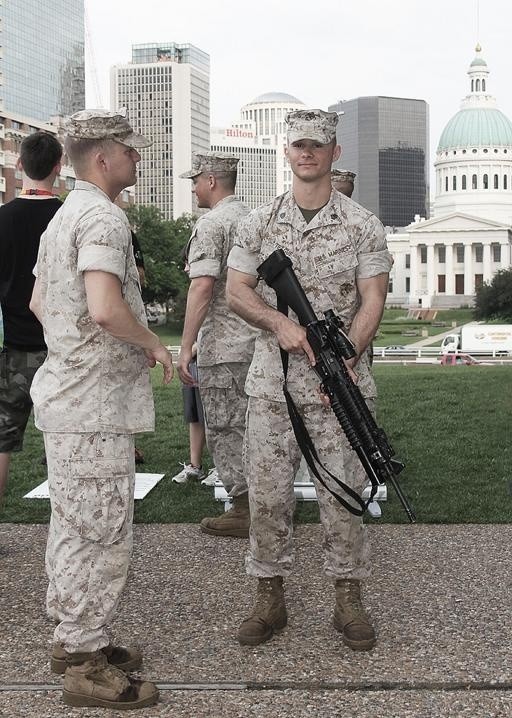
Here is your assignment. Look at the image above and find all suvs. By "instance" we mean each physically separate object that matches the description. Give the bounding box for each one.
[440,353,479,366]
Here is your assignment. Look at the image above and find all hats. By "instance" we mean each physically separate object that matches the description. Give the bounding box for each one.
[177,153,240,178]
[284,109,338,145]
[68,107,153,149]
[330,168,355,184]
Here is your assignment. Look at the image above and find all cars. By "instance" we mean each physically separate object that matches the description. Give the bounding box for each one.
[379,345,415,353]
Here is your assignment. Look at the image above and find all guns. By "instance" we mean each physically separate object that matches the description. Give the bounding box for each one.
[256,249,416,524]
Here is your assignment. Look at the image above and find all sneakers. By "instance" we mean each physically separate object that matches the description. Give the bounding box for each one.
[173,464,206,484]
[201,467,224,487]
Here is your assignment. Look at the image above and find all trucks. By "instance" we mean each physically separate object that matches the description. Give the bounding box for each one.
[441,322,512,357]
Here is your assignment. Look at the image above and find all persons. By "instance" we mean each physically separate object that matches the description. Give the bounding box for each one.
[177,150,260,539]
[327,168,375,367]
[2,131,65,504]
[224,107,397,654]
[171,241,223,490]
[41,230,148,465]
[28,106,173,712]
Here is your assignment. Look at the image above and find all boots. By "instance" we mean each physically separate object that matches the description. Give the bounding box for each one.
[238,576,287,644]
[62,651,159,709]
[52,640,140,673]
[334,580,374,650]
[199,493,250,538]
